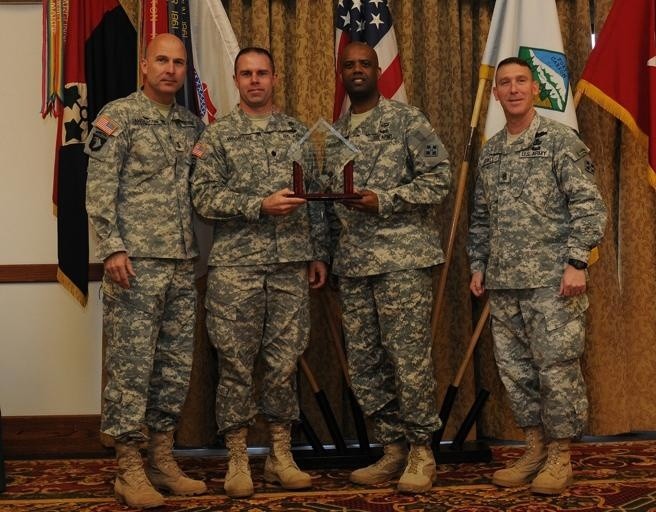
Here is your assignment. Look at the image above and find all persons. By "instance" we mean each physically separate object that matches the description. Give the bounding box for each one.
[190,48,333,498]
[322,40,452,493]
[85,32,207,509]
[465,58,607,495]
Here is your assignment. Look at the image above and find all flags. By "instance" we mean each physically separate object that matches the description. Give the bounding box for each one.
[481,0,600,268]
[333,0,409,122]
[41,0,241,306]
[189,0,240,281]
[576,0,656,190]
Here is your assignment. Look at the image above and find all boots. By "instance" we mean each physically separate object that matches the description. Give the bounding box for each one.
[113,430,207,509]
[350,441,408,485]
[264,420,312,490]
[532,438,573,494]
[492,425,548,487]
[397,444,437,495]
[222,427,255,499]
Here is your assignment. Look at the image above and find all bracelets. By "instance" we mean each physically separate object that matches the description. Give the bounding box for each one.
[568,258,587,269]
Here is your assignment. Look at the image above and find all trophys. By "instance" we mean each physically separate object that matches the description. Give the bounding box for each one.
[286,117,363,202]
